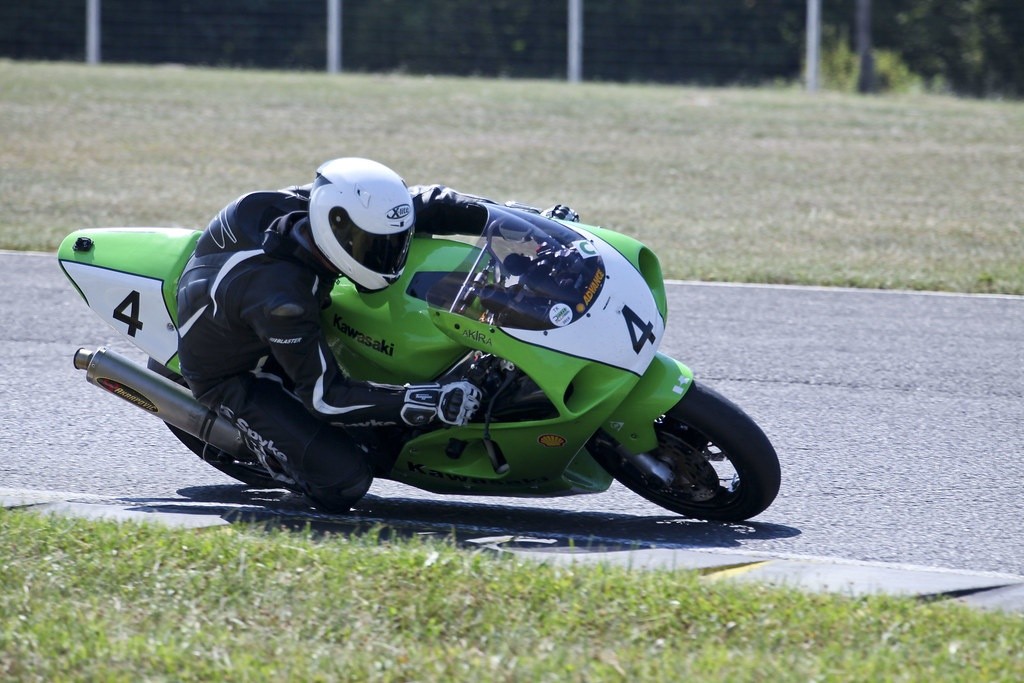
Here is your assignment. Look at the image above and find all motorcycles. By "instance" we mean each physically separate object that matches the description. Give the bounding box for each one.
[57,201,782,525]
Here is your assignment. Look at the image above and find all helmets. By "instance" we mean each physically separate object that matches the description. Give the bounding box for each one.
[308,157,415,293]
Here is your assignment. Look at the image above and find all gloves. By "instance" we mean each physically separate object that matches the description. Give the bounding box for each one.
[499,200,581,244]
[400,378,481,427]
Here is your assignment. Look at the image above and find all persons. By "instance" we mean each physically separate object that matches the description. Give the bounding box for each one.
[176,156,580,515]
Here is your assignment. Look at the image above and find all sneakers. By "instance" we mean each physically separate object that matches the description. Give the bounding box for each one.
[236,429,304,497]
